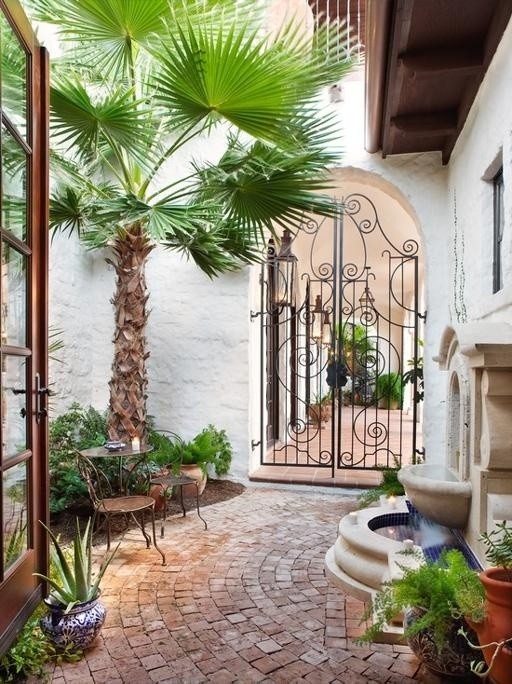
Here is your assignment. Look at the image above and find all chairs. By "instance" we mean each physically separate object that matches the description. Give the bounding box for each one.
[142,430,207,537]
[73,448,168,567]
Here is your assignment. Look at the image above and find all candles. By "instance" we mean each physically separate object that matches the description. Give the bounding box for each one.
[132,436,141,450]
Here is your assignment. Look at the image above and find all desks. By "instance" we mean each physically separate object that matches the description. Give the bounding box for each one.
[79,444,154,531]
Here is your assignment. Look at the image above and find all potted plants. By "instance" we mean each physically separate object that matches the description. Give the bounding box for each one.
[352,520,512,684]
[31,516,121,653]
[151,424,233,497]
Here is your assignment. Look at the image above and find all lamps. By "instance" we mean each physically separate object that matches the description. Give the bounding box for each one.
[322,310,333,345]
[311,295,325,339]
[275,229,299,308]
